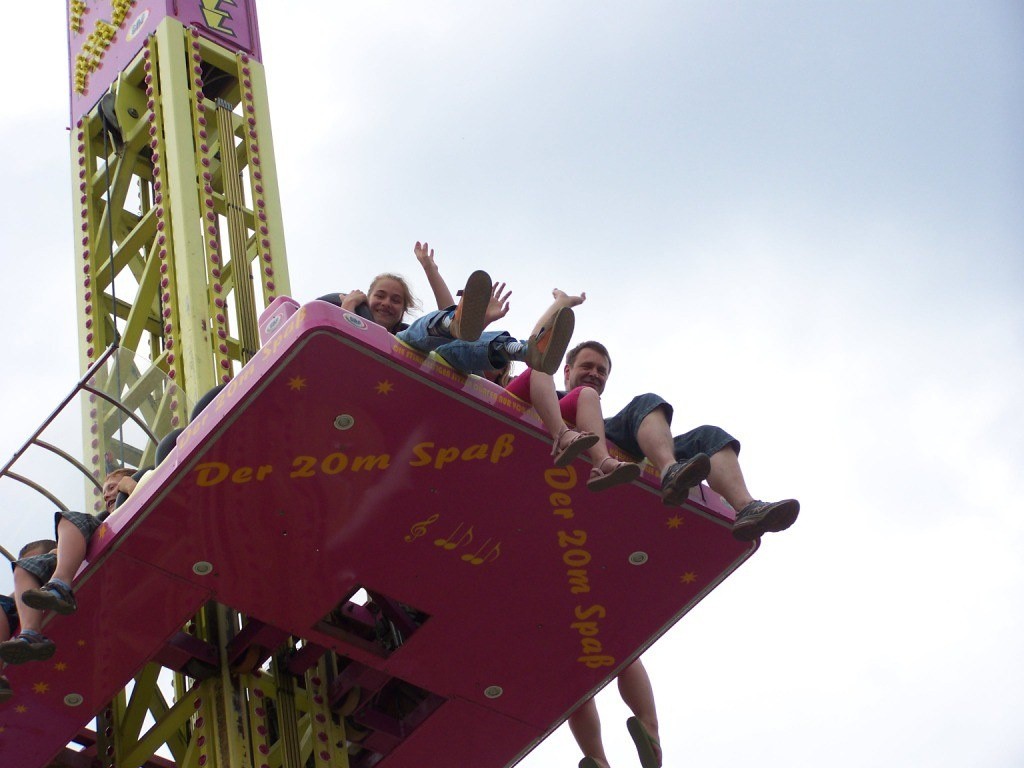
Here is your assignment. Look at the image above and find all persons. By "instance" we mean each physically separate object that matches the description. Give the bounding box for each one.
[338,240,576,375]
[565,655,662,768]
[415,240,637,490]
[532,289,800,542]
[0,466,137,664]
[0,539,58,643]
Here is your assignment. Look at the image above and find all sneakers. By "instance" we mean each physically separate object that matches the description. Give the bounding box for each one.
[450,270,493,342]
[526,305,575,376]
[734,499,801,540]
[660,452,710,506]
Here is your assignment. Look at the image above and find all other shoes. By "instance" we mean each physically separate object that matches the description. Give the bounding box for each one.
[0,675,13,700]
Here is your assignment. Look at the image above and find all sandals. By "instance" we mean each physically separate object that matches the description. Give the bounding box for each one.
[22,582,77,615]
[588,455,642,493]
[549,427,600,468]
[0,633,56,664]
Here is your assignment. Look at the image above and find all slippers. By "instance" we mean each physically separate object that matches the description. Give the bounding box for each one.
[578,756,610,768]
[626,716,663,768]
